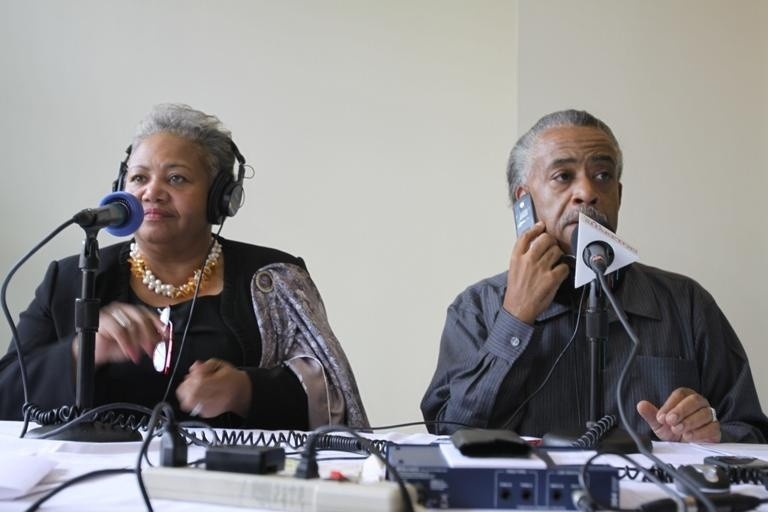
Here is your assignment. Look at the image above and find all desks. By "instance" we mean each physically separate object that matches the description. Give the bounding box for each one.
[0,418,747,511]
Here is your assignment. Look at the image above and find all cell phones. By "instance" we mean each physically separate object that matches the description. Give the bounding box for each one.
[512,194,540,243]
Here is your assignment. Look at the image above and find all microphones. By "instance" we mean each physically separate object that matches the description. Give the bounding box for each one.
[567,223,612,273]
[75,189,145,238]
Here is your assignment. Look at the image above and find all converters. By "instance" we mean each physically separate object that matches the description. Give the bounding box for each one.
[204,441,287,473]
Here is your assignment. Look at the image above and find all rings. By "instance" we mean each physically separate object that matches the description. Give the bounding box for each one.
[111,308,133,328]
[190,402,204,416]
[710,407,718,422]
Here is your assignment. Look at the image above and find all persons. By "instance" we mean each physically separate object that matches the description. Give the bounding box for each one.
[420,108,768,444]
[0,103,372,431]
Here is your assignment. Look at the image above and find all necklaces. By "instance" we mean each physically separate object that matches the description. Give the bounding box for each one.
[126,237,223,298]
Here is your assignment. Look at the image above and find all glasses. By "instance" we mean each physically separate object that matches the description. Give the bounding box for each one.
[153,306,173,375]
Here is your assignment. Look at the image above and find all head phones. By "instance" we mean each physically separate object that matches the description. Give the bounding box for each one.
[109,126,246,224]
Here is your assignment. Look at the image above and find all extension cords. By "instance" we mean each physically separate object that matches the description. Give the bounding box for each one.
[143,456,418,512]
